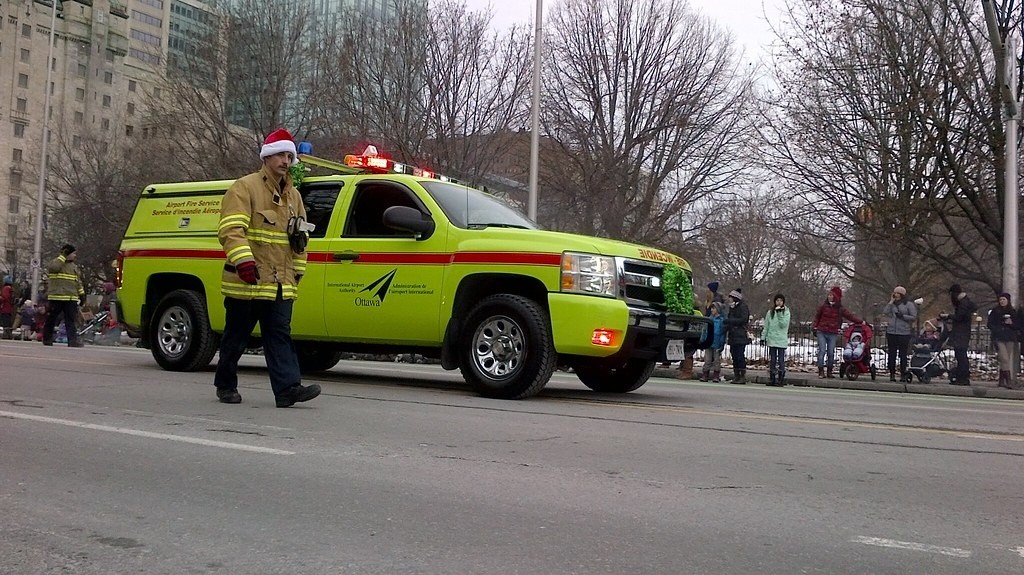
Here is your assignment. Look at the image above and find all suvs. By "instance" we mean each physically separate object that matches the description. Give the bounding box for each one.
[112,144,716,402]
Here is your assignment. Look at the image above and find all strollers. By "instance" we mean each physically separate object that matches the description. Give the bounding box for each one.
[903,319,958,383]
[839,322,876,381]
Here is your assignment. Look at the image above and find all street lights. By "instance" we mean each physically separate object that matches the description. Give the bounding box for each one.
[975,316,983,344]
[914,297,923,337]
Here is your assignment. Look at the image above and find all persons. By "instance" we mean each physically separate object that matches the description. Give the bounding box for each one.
[213,128,321,408]
[656,281,750,384]
[884,287,917,382]
[987,293,1024,389]
[813,286,866,379]
[914,317,941,349]
[844,332,865,359]
[940,283,976,385]
[0,245,117,348]
[760,293,791,386]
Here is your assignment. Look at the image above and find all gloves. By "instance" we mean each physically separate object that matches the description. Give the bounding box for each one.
[868,325,872,327]
[78,294,86,306]
[775,306,782,313]
[61,249,68,258]
[895,310,903,320]
[941,314,949,319]
[813,331,818,337]
[237,261,260,285]
[294,274,302,285]
[759,340,765,347]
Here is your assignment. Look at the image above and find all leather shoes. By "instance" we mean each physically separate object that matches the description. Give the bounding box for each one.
[44,343,53,346]
[275,384,321,408]
[216,388,242,403]
[68,341,84,347]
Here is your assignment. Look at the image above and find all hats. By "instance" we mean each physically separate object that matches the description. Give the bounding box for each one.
[707,281,742,314]
[774,294,785,303]
[260,128,296,166]
[61,244,76,256]
[3,275,13,284]
[829,283,1011,302]
[24,300,32,307]
[105,283,115,290]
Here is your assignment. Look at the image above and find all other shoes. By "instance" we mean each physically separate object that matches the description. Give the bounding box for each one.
[28,331,38,339]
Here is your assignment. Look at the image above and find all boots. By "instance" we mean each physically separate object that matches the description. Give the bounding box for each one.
[658,357,835,388]
[889,362,1014,390]
